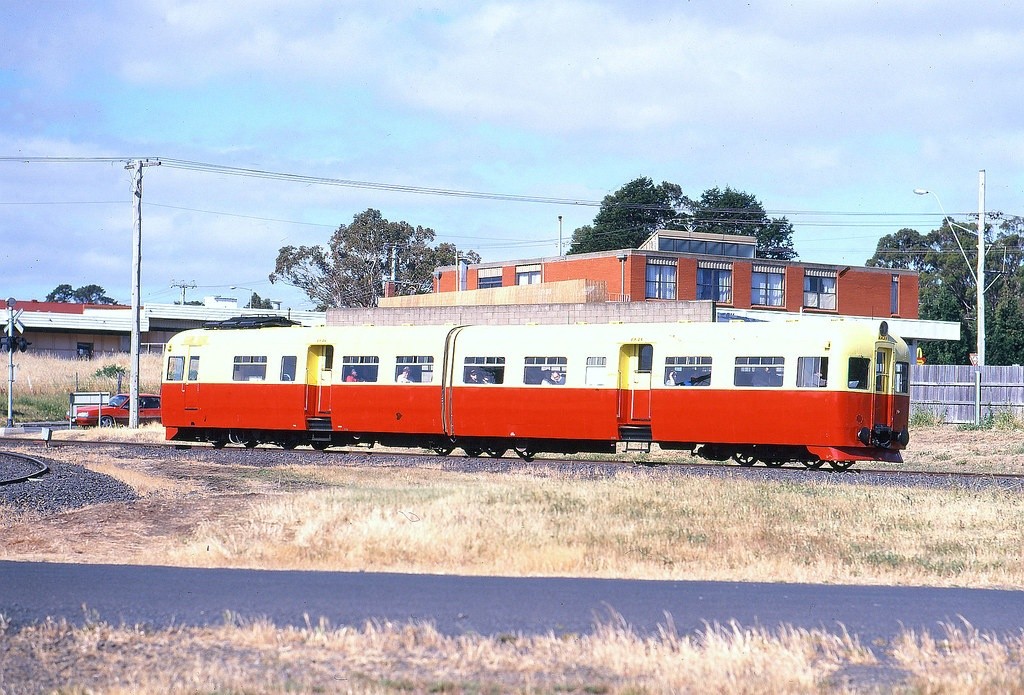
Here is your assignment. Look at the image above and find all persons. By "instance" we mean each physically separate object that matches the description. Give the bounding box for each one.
[340,355,871,391]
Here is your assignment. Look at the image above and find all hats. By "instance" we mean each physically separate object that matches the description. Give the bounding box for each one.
[469,369,476,373]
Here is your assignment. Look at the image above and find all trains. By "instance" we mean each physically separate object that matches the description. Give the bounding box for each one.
[157,310,915,472]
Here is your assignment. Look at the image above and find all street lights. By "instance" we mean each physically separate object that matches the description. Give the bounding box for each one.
[911,188,977,284]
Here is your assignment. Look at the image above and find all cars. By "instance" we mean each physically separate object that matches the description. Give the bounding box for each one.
[64,391,163,430]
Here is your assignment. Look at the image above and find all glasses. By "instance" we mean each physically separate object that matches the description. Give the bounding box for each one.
[471,374,477,376]
